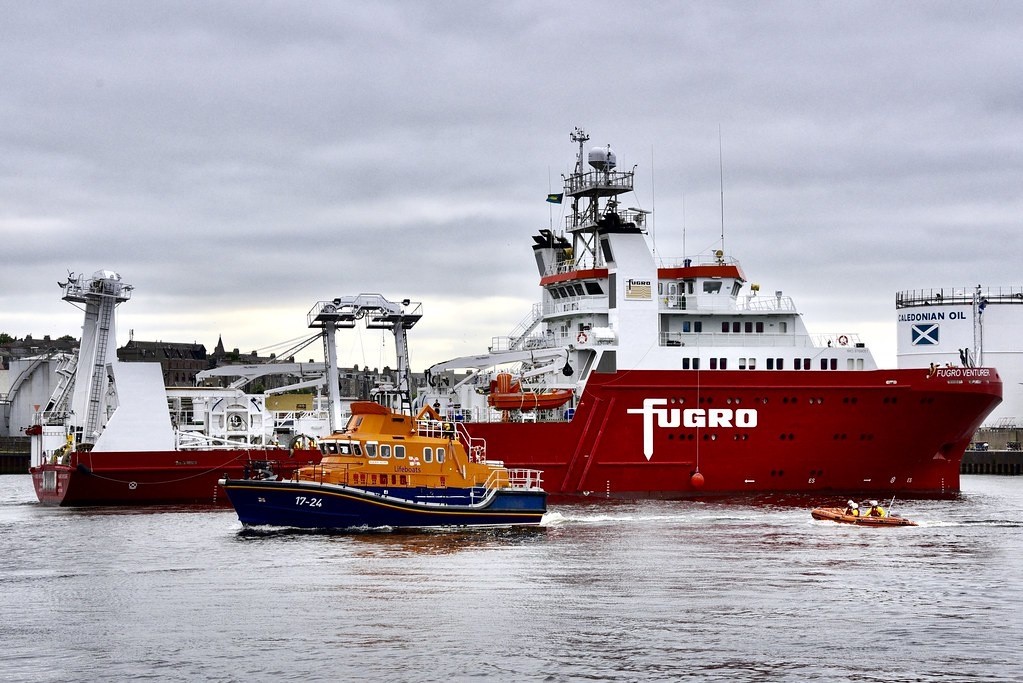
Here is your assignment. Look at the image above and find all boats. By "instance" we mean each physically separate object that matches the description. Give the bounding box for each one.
[811,507,920,526]
[25,126,1003,508]
[487,389,572,413]
[218,392,547,534]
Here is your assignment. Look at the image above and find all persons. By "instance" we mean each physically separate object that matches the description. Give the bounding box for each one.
[1007,442,1021,451]
[437,423,455,440]
[433,399,440,414]
[865,500,885,517]
[845,500,859,516]
[447,398,454,419]
[983,442,988,451]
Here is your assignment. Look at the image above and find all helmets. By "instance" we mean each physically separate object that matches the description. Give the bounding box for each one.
[870,500,878,506]
[847,500,854,506]
[851,503,858,508]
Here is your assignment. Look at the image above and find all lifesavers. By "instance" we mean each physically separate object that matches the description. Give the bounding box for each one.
[839,335,848,345]
[577,334,587,344]
[716,250,723,258]
[473,445,485,461]
